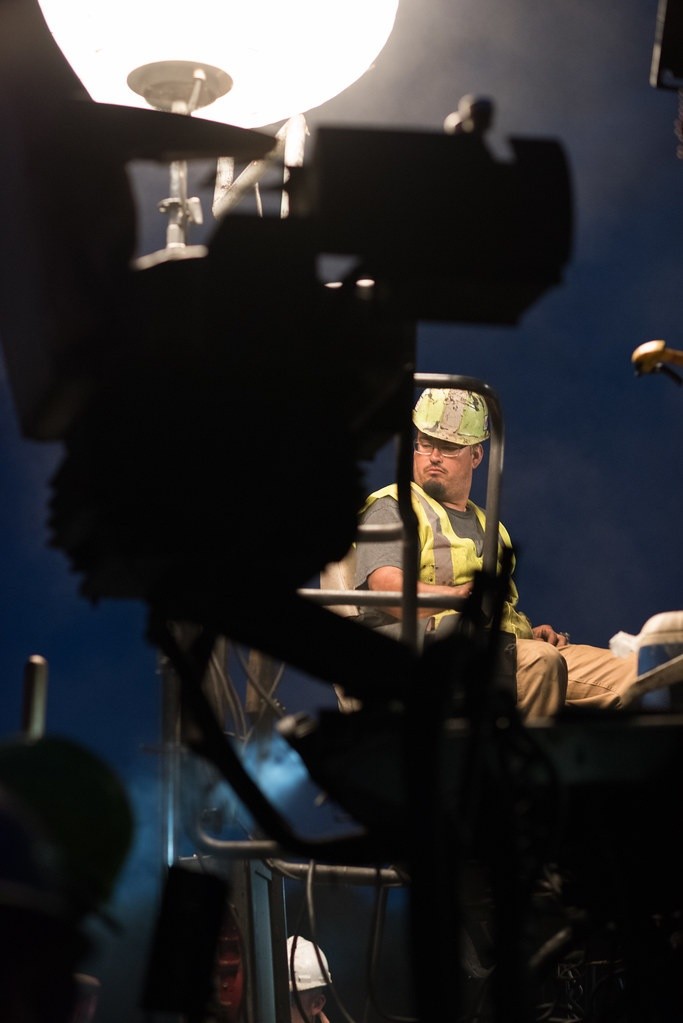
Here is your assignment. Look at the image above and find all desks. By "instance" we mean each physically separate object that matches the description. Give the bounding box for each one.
[438,711,683,1023]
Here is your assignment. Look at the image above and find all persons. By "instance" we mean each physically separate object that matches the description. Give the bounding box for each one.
[287,936,332,1023]
[321,388,611,726]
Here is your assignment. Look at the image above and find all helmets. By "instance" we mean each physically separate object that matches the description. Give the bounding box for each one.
[412,388,489,445]
[286,936,332,991]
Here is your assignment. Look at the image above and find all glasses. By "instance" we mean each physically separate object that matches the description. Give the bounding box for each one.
[416,441,466,457]
